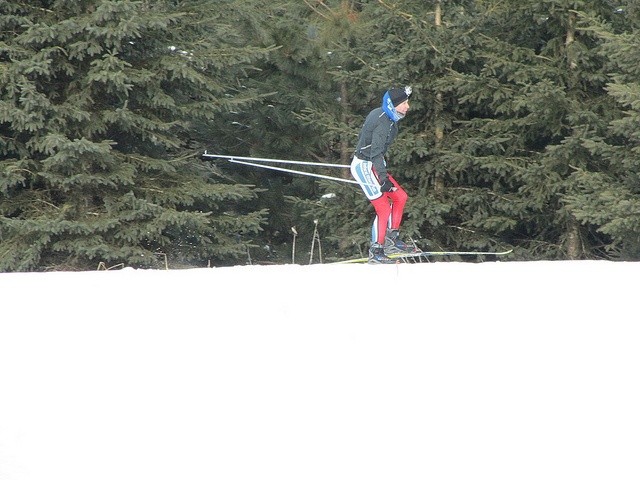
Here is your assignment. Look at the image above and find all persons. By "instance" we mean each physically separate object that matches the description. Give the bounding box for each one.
[351,85,416,264]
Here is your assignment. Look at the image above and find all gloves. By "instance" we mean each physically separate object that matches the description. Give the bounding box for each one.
[380,178,395,193]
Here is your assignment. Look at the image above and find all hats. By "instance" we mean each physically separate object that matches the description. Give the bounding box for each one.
[388,86,408,107]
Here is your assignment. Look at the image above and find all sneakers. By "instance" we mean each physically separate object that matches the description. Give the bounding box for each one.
[385,228,407,249]
[367,242,396,264]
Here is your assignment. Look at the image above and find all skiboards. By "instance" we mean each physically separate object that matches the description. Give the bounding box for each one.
[333,249,514,263]
